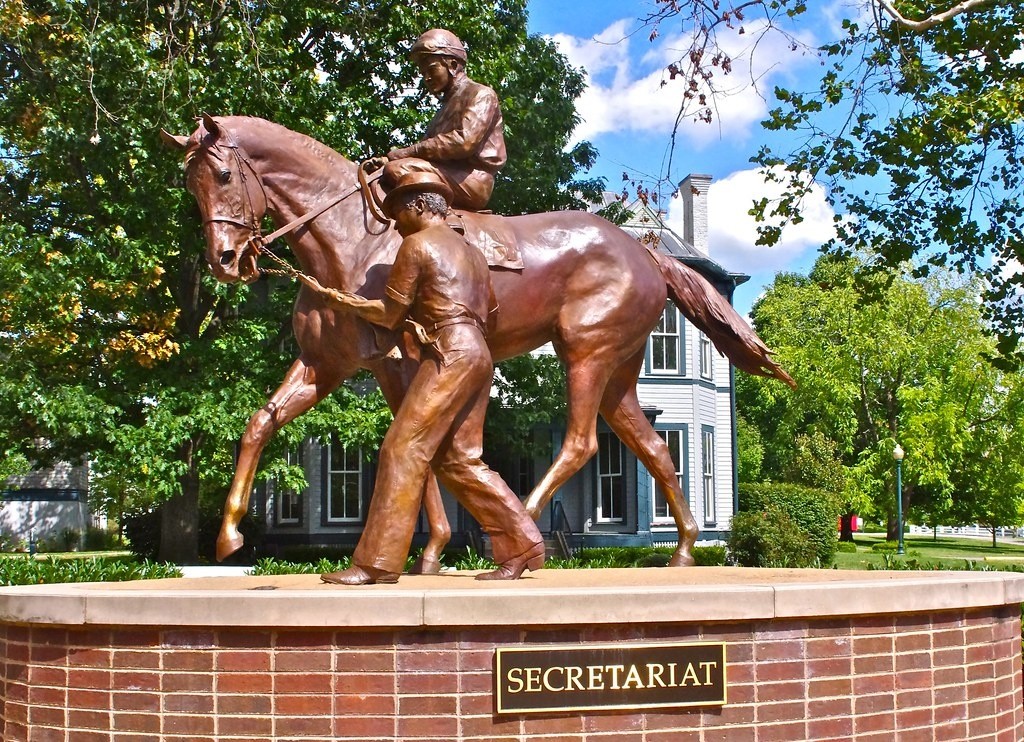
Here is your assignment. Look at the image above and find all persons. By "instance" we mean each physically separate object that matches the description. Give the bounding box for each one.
[320,172,545,585]
[365,29,508,212]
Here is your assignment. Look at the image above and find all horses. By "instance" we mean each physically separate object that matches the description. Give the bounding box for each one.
[159,110,798,577]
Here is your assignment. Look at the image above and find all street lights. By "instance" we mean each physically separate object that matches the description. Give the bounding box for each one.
[893,444,905,555]
